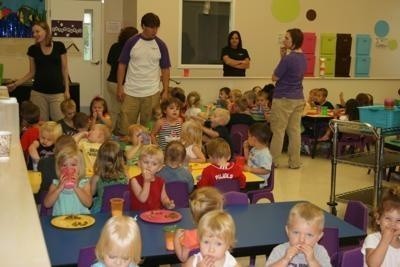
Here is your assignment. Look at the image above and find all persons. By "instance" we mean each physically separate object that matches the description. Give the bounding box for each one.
[72,111,90,144]
[394,89,400,106]
[242,91,256,110]
[339,91,373,107]
[261,84,275,99]
[217,88,229,107]
[185,91,212,119]
[232,98,247,113]
[361,188,399,267]
[356,93,369,106]
[106,26,140,128]
[316,88,333,109]
[146,104,163,137]
[57,98,78,136]
[90,141,130,216]
[265,100,272,122]
[317,102,359,142]
[253,86,262,96]
[171,86,188,117]
[186,210,240,266]
[157,139,195,195]
[250,91,270,115]
[197,137,247,189]
[239,122,273,203]
[125,124,151,165]
[150,98,182,150]
[308,88,319,105]
[90,216,145,267]
[191,108,235,157]
[27,121,64,170]
[113,12,171,137]
[225,90,240,111]
[43,145,93,217]
[19,100,41,164]
[221,30,251,76]
[6,21,71,121]
[266,202,332,266]
[72,123,112,177]
[179,119,205,163]
[130,144,175,211]
[36,135,77,202]
[173,186,225,263]
[269,27,308,171]
[87,95,113,131]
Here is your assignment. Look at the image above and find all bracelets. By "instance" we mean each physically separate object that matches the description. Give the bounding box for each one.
[14,82,18,88]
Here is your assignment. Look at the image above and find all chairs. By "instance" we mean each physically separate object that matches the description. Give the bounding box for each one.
[231,131,242,162]
[123,191,130,211]
[40,191,51,216]
[100,185,129,212]
[216,179,240,192]
[362,135,370,152]
[340,201,368,255]
[318,228,340,267]
[223,192,255,267]
[342,248,363,267]
[231,124,248,152]
[118,149,126,162]
[165,181,189,208]
[247,163,274,204]
[337,134,361,156]
[78,247,96,267]
[189,248,199,257]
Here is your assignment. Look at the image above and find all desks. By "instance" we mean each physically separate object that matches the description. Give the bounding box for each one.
[306,114,338,158]
[168,77,400,104]
[40,201,366,266]
[27,163,265,204]
[2,82,80,111]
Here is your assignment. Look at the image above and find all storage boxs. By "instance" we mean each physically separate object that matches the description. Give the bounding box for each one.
[358,106,400,128]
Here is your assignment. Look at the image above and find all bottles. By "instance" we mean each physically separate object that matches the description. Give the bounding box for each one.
[384,97,394,112]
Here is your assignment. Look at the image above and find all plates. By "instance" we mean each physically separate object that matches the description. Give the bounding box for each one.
[139,209,183,223]
[50,214,96,228]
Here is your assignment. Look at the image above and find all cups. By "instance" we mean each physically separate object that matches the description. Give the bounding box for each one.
[321,106,328,115]
[137,131,150,144]
[61,166,77,188]
[109,197,125,216]
